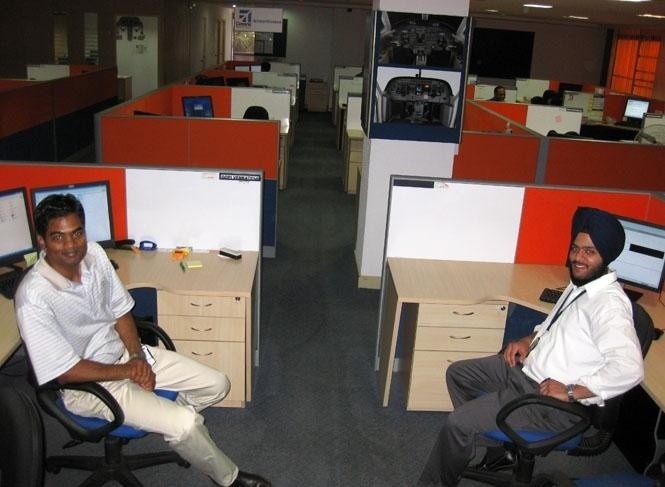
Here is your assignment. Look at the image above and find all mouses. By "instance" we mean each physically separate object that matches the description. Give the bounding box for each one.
[652,327,664,340]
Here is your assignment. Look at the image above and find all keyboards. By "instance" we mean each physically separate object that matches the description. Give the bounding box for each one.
[540,287,562,303]
[0,268,25,300]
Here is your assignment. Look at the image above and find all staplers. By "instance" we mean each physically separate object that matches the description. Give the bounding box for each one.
[115,239,135,250]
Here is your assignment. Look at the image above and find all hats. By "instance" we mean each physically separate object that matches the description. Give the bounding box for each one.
[571,207,625,265]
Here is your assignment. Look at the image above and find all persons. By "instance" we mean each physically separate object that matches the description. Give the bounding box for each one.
[259,61,271,72]
[414,206,646,485]
[241,103,269,120]
[487,85,508,101]
[11,191,271,485]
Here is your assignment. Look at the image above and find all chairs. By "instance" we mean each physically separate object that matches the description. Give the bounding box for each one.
[15,316,193,487]
[455,300,658,487]
[241,107,268,119]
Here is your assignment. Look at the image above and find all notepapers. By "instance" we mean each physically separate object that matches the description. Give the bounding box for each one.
[187,260,203,269]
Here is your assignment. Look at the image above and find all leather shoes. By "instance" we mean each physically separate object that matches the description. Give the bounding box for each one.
[230,470,272,487]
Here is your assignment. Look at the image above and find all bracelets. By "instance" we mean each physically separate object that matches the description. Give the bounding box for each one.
[567,384,575,403]
[128,350,146,358]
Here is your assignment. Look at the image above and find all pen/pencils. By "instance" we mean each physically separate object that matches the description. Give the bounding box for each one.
[180,262,186,273]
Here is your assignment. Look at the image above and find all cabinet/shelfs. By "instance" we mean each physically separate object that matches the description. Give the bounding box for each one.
[304,71,328,110]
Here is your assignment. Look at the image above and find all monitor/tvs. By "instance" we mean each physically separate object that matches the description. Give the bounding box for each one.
[623,98,651,124]
[234,65,249,72]
[227,77,250,87]
[29,180,115,249]
[196,76,225,86]
[250,64,261,72]
[607,214,665,302]
[181,96,214,118]
[0,187,36,275]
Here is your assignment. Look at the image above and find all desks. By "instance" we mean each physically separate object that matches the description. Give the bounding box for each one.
[277,123,290,194]
[376,252,665,476]
[341,127,368,199]
[0,241,263,421]
